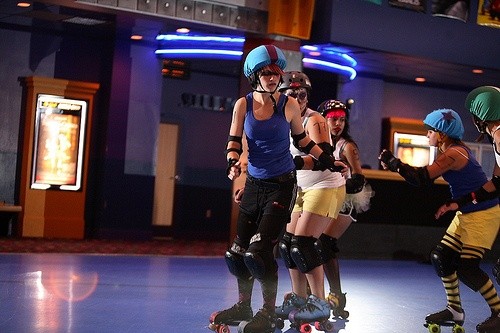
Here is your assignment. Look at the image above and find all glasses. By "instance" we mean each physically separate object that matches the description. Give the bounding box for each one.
[259,70,280,76]
[287,92,307,100]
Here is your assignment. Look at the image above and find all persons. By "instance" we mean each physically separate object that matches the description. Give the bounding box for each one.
[209,43,366,333]
[378,84,500,333]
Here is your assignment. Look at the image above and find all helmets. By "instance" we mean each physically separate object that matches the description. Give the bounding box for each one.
[277,71,311,92]
[317,100,350,120]
[244,45,287,78]
[423,109,464,141]
[465,86,500,122]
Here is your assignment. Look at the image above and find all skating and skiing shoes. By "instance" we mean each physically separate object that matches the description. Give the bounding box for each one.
[275,293,333,333]
[423,304,465,333]
[237,310,284,333]
[325,292,349,319]
[476,318,500,333]
[209,302,253,333]
